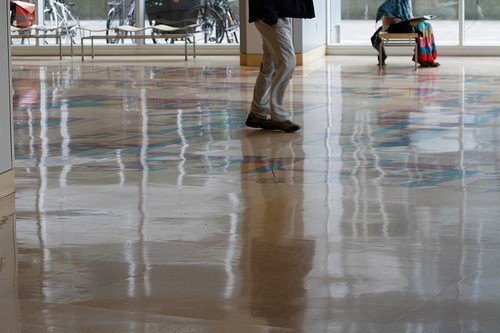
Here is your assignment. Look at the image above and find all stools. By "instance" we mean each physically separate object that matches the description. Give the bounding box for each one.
[378,31,418,72]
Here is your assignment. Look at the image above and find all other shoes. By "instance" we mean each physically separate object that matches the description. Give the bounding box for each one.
[412,56,428,67]
[426,61,440,67]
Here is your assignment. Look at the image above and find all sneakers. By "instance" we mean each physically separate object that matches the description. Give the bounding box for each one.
[267,119,301,133]
[244,113,267,129]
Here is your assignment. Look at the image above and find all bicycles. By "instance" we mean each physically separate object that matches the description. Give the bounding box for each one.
[105,0,136,44]
[42,0,80,44]
[181,0,239,43]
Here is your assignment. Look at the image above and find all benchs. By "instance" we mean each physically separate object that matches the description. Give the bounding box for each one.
[11,22,76,60]
[76,24,201,62]
